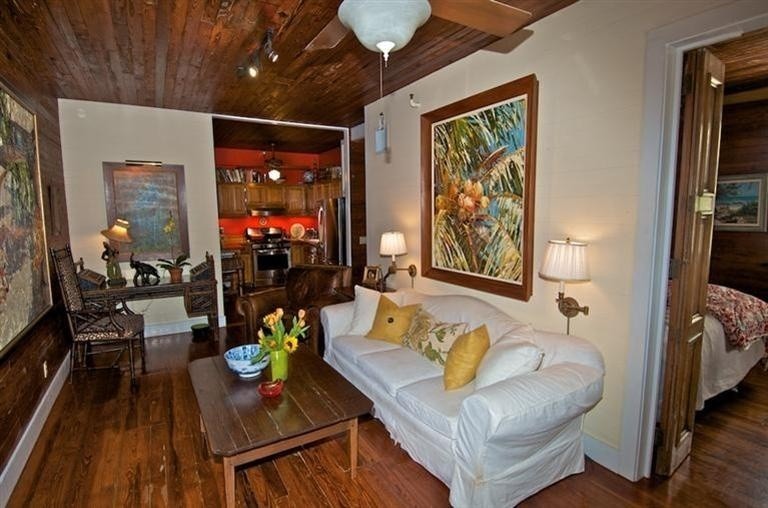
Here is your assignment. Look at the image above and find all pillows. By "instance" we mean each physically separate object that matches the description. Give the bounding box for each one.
[349,285,545,391]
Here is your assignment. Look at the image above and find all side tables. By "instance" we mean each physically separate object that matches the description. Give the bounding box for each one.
[336,286,398,300]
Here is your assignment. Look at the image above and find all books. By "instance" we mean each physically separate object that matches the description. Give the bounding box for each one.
[216,167,268,184]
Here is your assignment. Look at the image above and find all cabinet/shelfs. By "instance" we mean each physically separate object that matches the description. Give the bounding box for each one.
[221,238,253,289]
[219,180,341,217]
[292,244,305,268]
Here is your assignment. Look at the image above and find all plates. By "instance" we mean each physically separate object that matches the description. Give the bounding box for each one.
[290,221,305,239]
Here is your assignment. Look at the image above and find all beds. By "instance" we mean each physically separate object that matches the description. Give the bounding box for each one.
[659,279,768,417]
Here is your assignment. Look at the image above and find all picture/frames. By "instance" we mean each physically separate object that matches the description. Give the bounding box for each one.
[0,81,55,361]
[420,73,538,301]
[713,173,768,232]
[101,159,190,262]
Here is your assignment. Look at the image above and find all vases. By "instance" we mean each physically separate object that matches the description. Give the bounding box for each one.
[270,350,289,382]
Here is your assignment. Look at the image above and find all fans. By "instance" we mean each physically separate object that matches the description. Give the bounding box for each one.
[305,0,531,52]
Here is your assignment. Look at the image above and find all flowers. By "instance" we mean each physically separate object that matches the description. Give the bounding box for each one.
[250,308,310,354]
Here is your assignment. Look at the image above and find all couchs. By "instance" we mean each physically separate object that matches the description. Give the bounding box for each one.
[235,265,355,357]
[320,287,604,507]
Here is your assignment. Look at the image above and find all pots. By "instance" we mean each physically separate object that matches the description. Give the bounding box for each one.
[248,233,265,243]
[262,231,281,241]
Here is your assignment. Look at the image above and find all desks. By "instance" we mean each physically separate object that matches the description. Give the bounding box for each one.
[72,252,221,343]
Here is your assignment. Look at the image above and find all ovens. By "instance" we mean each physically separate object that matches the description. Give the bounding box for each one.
[251,241,293,288]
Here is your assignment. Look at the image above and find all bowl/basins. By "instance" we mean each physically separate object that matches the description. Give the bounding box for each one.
[222,343,270,379]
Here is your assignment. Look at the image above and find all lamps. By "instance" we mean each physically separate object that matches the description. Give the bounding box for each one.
[265,30,280,63]
[539,238,590,316]
[101,217,132,285]
[247,49,264,77]
[379,231,416,277]
[337,1,432,70]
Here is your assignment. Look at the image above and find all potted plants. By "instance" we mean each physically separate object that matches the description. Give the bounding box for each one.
[156,255,190,284]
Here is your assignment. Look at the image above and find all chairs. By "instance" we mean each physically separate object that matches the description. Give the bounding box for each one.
[51,244,147,385]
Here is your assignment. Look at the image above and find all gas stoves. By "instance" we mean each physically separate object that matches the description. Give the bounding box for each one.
[245,238,292,250]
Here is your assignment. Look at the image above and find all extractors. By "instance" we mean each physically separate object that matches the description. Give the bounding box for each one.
[248,207,286,217]
